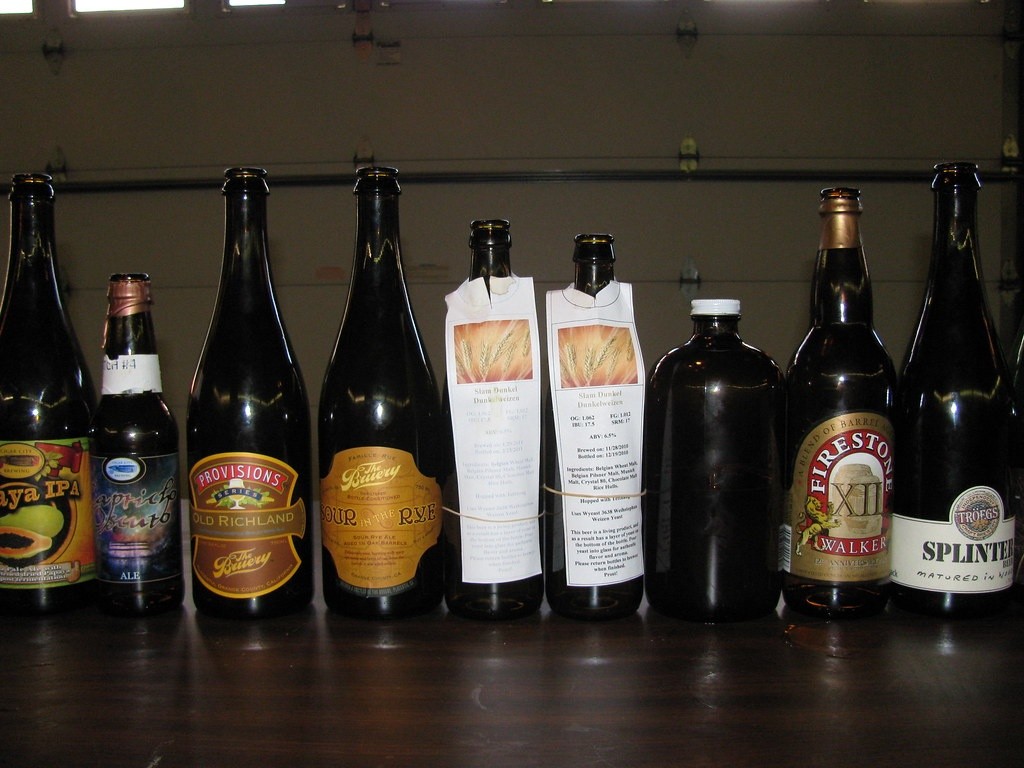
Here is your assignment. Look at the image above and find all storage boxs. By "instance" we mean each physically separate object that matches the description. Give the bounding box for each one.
[3,154,1022,630]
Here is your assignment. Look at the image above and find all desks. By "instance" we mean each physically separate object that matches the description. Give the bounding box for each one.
[2,497,1024,768]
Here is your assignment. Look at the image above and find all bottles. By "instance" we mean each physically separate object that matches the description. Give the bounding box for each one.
[889,159,1021,619]
[780,186,903,619]
[641,296,798,621]
[1,170,103,616]
[448,216,554,623]
[182,169,323,620]
[531,231,648,619]
[314,165,448,624]
[89,272,185,618]
